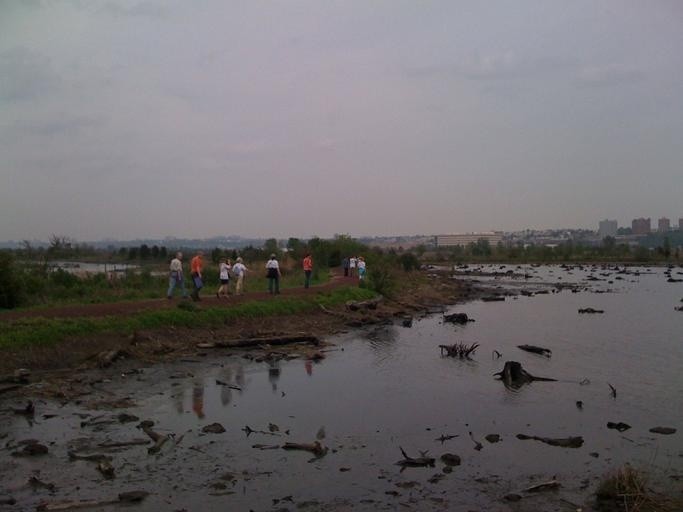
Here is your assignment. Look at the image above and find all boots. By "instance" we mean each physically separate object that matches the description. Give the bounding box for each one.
[190,293,203,302]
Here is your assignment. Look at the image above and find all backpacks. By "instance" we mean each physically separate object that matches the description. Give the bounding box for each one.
[234,264,241,276]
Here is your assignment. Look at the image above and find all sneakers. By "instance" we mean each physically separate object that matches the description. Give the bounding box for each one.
[166,295,188,299]
[216,292,220,299]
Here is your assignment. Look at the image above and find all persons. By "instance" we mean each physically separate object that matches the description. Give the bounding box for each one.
[356,257,365,280]
[232,256,252,296]
[265,252,281,296]
[302,253,313,288]
[348,255,357,278]
[216,257,232,299]
[167,360,314,423]
[188,250,205,301]
[164,251,188,300]
[343,256,349,277]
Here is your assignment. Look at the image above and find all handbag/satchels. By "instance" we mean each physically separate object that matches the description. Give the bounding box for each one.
[219,263,229,280]
[195,277,204,289]
[265,267,279,279]
